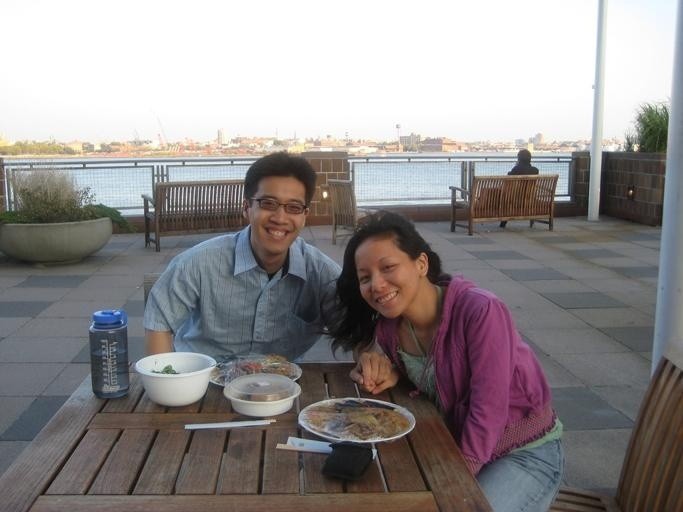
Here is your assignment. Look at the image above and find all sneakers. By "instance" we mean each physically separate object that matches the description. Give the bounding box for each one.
[500,221,507,227]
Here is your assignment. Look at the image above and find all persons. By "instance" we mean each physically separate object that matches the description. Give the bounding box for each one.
[143,152,347,363]
[499,149,539,227]
[329,211,566,512]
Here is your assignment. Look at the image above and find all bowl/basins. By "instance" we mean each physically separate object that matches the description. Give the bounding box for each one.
[135,351,217,406]
[222,372,302,418]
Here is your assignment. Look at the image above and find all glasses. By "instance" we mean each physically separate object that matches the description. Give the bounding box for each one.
[251,198,307,215]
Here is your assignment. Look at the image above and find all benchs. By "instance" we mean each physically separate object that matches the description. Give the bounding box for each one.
[139,178,246,251]
[449,172,559,236]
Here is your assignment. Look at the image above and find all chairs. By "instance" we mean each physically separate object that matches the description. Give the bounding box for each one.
[546,355,683,512]
[327,178,379,245]
[145,273,161,306]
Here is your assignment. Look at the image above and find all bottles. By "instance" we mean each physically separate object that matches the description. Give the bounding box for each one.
[88,309,130,400]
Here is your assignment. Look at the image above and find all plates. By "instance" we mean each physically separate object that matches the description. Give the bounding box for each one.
[297,396,416,445]
[210,357,303,388]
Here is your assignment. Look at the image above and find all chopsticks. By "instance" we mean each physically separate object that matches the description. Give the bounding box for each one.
[184,418,276,430]
[275,443,377,460]
